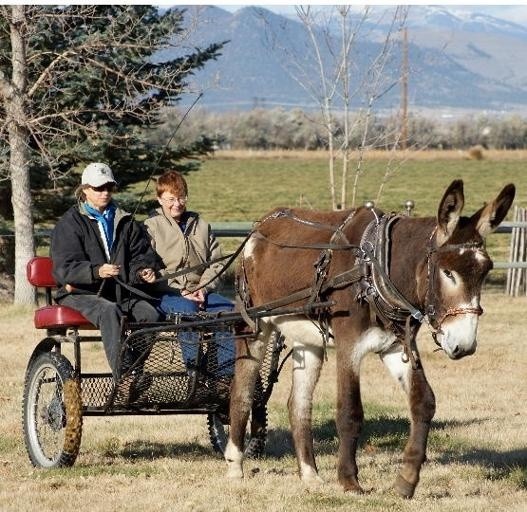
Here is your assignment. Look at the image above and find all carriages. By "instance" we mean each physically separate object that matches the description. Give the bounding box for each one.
[21,177,517,502]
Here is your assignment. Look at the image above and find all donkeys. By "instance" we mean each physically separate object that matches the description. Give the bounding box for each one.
[223,176,519,503]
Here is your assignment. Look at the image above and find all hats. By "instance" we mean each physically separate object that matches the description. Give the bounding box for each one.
[82,163,118,187]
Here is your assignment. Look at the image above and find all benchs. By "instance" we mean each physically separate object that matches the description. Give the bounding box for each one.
[27,257,100,331]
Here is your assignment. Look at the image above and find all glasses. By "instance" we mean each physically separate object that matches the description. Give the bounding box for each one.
[161,195,188,205]
[90,185,114,192]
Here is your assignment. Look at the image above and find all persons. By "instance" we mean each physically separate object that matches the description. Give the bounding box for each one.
[138,169,237,408]
[49,160,164,408]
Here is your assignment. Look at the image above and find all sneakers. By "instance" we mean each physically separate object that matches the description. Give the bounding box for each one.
[188,379,230,405]
[122,372,152,404]
